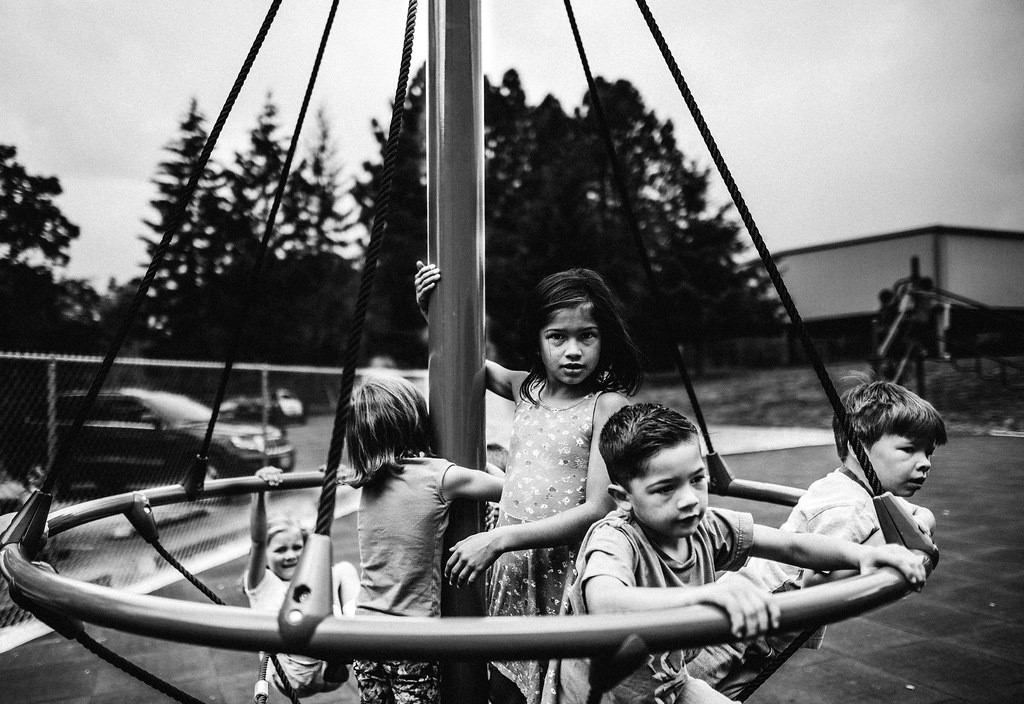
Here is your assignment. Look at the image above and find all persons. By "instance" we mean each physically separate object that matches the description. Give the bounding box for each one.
[242,262,948,704]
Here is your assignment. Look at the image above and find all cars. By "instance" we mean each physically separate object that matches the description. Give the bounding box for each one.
[14,388,295,503]
[219,384,307,425]
